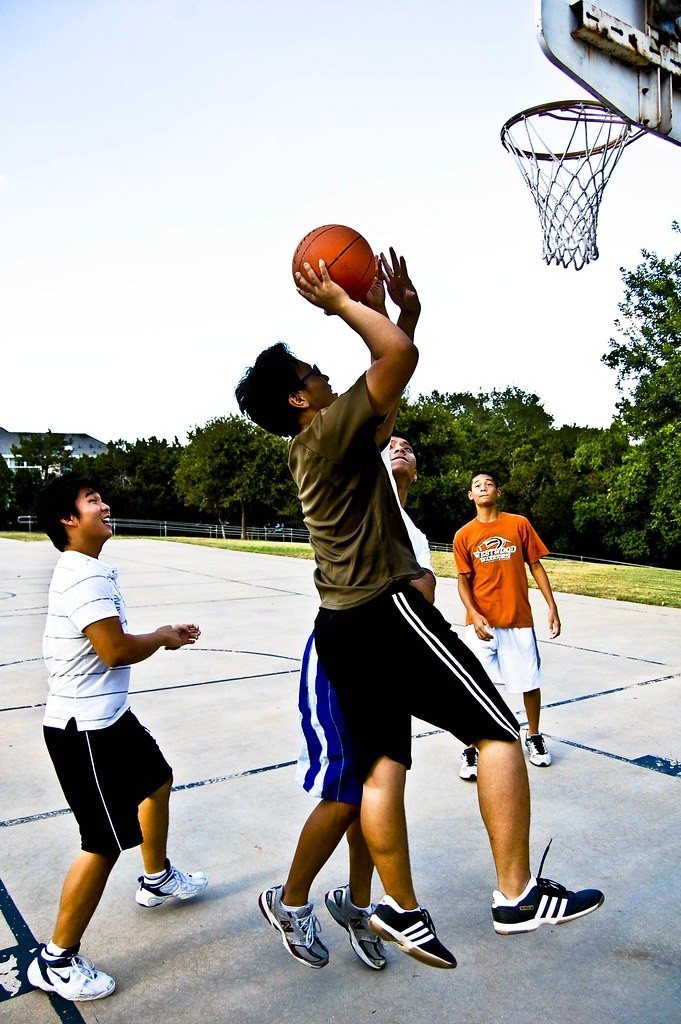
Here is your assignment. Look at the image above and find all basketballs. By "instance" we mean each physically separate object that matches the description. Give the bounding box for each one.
[293,224,377,306]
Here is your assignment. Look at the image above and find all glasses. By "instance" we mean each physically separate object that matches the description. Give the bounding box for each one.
[301,364,321,382]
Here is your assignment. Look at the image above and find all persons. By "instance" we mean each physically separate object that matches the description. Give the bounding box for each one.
[257,247,422,971]
[234,246,605,968]
[452,470,562,780]
[27,471,209,1003]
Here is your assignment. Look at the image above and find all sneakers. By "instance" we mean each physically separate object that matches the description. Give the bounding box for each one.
[369,895,457,969]
[525,732,552,767]
[460,747,478,781]
[27,942,116,1002]
[135,858,208,908]
[325,883,386,970]
[491,839,604,935]
[258,885,329,969]
